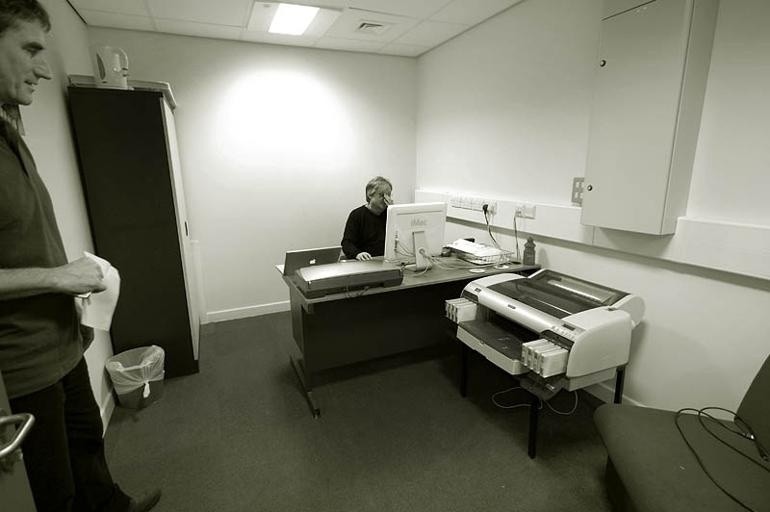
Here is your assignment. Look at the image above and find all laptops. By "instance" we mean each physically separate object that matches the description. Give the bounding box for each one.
[274,246,342,276]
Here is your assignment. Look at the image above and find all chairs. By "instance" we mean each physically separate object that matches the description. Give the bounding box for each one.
[594,354,770,512]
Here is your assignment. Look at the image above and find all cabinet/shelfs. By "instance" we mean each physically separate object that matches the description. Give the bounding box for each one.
[580,0,719,235]
[67,85,201,378]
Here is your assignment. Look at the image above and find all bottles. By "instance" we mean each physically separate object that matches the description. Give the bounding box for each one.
[522,236,537,266]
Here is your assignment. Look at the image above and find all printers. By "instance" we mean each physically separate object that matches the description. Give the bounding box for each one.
[445,268,645,401]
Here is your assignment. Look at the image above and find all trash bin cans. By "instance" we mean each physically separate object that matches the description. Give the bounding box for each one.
[106,345,167,411]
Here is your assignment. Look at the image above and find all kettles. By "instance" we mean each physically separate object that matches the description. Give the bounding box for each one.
[96,46,130,91]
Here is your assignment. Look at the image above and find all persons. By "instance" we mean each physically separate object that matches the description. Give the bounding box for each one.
[1,0,161,512]
[341,176,393,261]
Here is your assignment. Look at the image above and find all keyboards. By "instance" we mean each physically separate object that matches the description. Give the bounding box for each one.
[384,257,440,266]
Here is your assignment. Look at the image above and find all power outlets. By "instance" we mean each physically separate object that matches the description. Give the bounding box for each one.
[516,203,536,218]
[450,195,497,213]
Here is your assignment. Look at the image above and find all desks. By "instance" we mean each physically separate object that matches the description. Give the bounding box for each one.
[274,254,540,419]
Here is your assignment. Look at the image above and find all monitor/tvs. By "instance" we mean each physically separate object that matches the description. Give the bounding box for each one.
[384,202,447,272]
